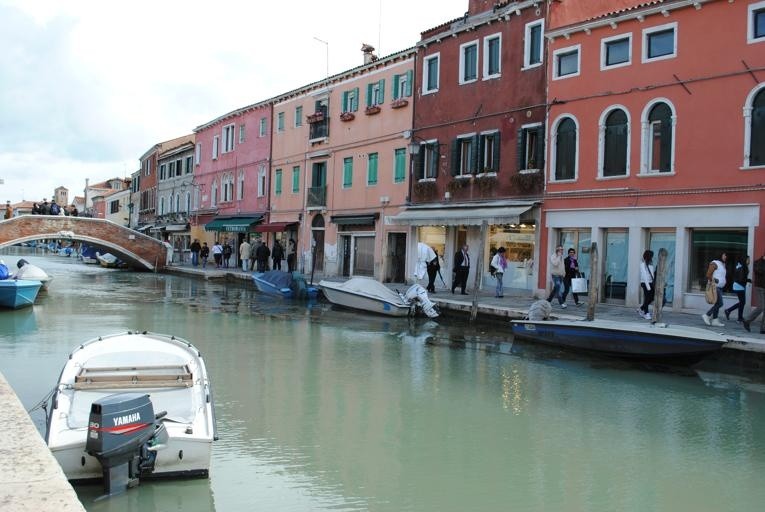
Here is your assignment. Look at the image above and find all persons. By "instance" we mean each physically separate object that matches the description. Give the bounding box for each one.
[30,199,78,217]
[490,246,506,298]
[562,248,586,307]
[701,250,728,327]
[724,255,755,324]
[4,203,13,219]
[424,246,441,294]
[742,252,765,334]
[157,237,297,275]
[546,245,568,310]
[637,249,655,321]
[451,245,470,296]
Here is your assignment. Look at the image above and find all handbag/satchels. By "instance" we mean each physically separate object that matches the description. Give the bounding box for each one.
[571,278,588,293]
[705,279,717,304]
[490,266,497,281]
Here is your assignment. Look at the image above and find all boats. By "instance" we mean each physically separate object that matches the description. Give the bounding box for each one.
[254,293,302,319]
[1,274,44,311]
[23,239,123,267]
[85,475,215,512]
[0,306,37,341]
[42,326,220,486]
[311,307,412,333]
[249,269,320,302]
[319,277,418,318]
[503,305,729,367]
[504,336,739,397]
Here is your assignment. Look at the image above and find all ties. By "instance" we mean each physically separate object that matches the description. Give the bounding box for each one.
[465,257,467,265]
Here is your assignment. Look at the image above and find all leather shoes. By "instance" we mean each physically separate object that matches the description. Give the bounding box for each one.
[431,291,436,293]
[461,293,468,295]
[452,288,454,294]
[427,287,430,292]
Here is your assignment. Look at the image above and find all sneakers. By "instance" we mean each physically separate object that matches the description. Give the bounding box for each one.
[637,307,651,320]
[576,301,585,307]
[760,330,765,334]
[561,304,567,308]
[494,296,503,297]
[702,309,750,331]
